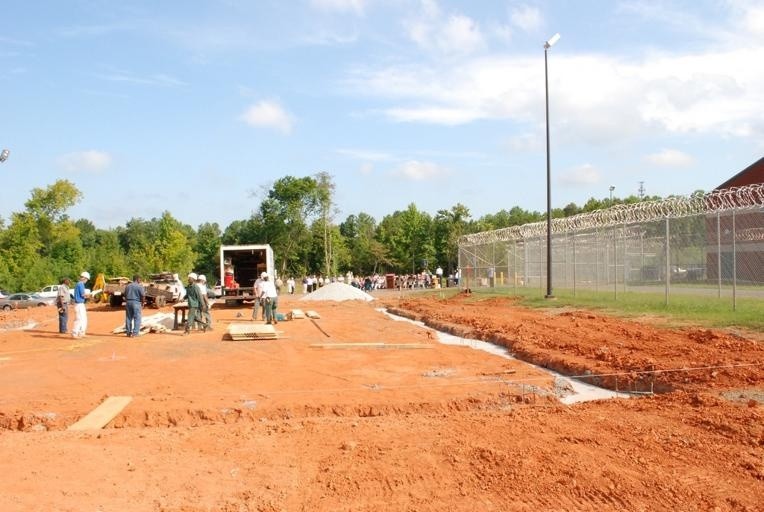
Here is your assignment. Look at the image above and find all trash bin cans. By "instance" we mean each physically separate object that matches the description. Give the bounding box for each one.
[446,277,455,288]
[386,274,395,289]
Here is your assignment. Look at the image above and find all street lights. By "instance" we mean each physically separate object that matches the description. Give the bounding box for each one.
[543,32,560,295]
[609,186,615,206]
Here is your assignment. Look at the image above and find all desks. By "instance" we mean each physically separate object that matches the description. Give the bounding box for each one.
[173,301,189,329]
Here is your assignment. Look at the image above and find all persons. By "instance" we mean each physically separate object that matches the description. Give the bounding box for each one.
[178,273,208,335]
[259,275,278,324]
[55,276,70,334]
[487,261,493,287]
[70,271,92,340]
[275,266,462,295]
[250,272,268,321]
[122,274,144,337]
[196,275,214,331]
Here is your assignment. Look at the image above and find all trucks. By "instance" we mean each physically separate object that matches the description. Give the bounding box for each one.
[220,244,274,306]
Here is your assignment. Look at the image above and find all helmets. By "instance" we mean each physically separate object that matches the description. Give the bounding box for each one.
[188,272,198,281]
[80,271,91,280]
[198,274,207,282]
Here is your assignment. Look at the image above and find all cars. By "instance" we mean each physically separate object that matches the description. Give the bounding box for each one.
[207,286,221,298]
[0,285,90,311]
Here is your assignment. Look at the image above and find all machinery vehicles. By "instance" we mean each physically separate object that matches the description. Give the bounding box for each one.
[91,271,132,304]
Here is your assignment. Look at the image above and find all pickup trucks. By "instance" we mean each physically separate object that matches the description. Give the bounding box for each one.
[149,279,186,302]
